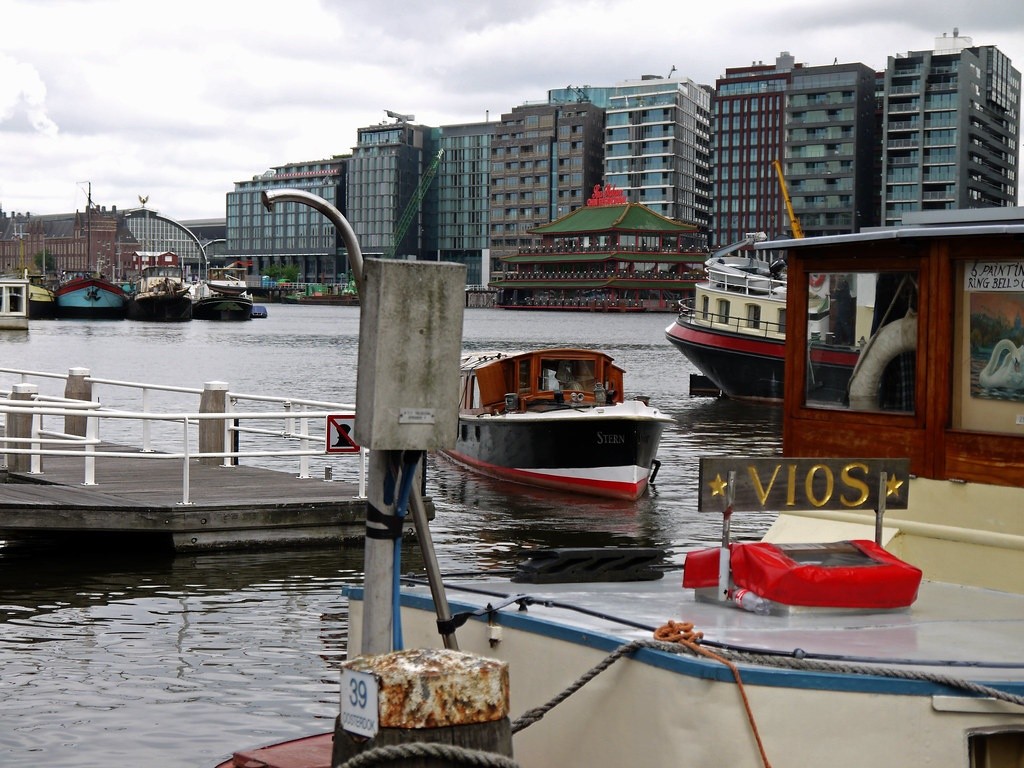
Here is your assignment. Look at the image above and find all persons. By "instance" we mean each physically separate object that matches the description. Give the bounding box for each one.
[554,367,583,392]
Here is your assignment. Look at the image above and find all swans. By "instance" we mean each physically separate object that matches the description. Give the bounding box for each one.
[979,339,1024,390]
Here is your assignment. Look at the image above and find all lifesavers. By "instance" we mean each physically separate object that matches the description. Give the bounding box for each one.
[842,312,931,420]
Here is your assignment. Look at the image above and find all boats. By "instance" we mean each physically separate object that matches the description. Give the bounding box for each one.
[0,180,267,323]
[665,230,859,408]
[438,346,679,501]
